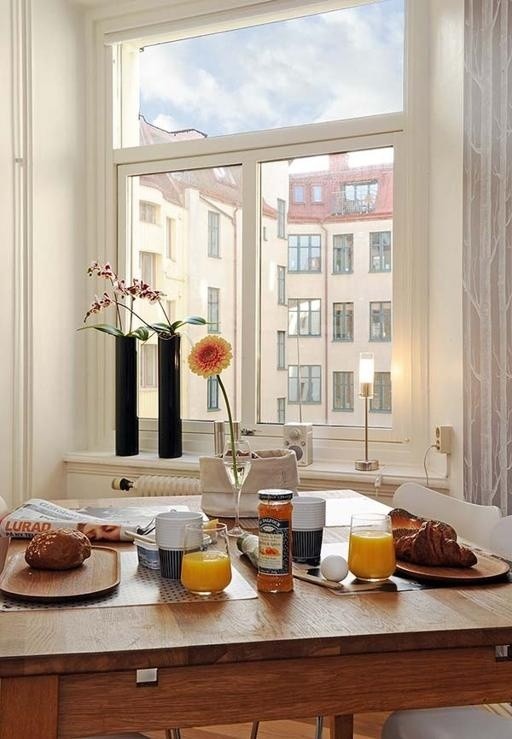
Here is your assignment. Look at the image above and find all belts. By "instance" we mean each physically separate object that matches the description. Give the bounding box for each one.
[221,440,252,540]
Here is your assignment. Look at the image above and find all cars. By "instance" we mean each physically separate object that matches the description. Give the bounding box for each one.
[347,511,397,582]
[179,523,232,595]
[292,497,326,564]
[154,511,203,578]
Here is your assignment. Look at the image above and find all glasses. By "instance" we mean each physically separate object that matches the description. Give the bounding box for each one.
[354,353,378,471]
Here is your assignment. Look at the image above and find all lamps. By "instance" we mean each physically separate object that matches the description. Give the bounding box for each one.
[382,481,512,739]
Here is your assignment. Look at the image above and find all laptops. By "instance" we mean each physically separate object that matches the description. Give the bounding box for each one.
[24,528,93,572]
[388,507,477,570]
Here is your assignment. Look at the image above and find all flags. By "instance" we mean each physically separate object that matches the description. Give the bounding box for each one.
[115,336,139,456]
[159,333,181,459]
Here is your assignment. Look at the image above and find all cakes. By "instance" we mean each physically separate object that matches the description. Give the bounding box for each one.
[257,488,295,595]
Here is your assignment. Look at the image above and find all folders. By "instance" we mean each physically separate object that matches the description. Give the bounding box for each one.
[113,475,202,497]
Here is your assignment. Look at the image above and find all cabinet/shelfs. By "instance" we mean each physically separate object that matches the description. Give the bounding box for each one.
[236,532,260,571]
[255,490,294,595]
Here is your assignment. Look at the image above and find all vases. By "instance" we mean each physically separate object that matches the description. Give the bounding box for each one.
[83,279,205,338]
[76,257,148,340]
[188,335,238,489]
